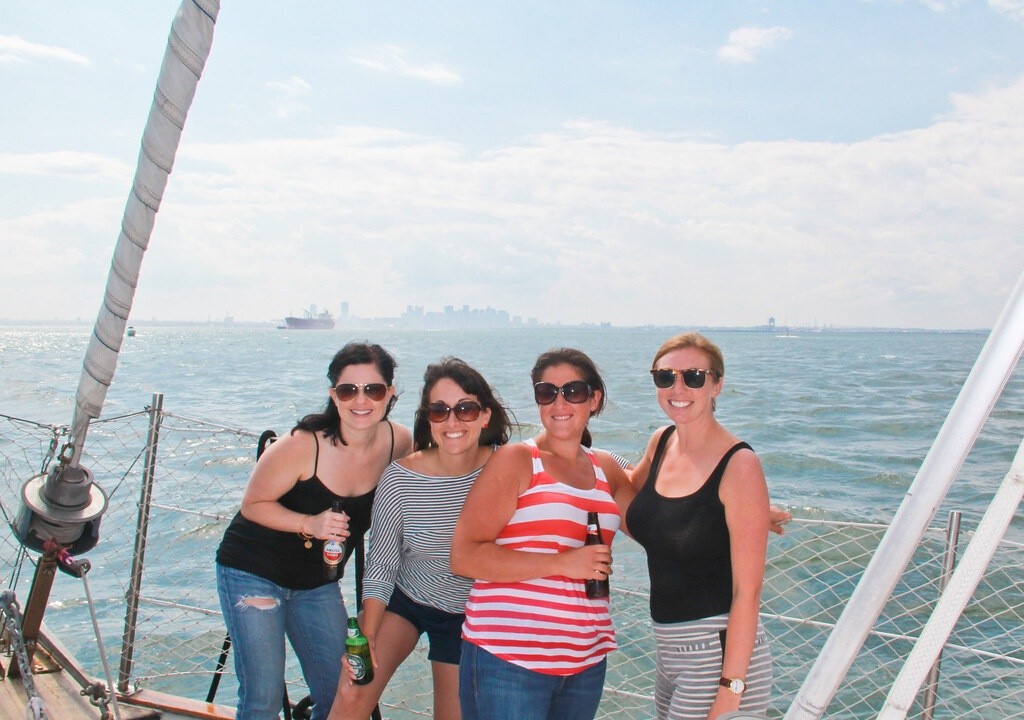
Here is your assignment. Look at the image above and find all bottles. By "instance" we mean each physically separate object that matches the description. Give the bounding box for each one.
[320,499,346,581]
[585,512,610,600]
[345,617,375,686]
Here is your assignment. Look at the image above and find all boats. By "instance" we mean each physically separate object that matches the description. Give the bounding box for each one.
[284,308,335,330]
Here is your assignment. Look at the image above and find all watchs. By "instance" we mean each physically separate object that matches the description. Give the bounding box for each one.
[719,677,747,695]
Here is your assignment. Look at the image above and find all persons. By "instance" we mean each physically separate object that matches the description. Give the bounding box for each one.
[326,358,634,720]
[449,348,794,720]
[215,341,413,720]
[621,333,771,720]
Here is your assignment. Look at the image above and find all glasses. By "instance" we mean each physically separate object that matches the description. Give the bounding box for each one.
[531,380,594,405]
[423,400,485,424]
[650,366,716,390]
[330,382,390,402]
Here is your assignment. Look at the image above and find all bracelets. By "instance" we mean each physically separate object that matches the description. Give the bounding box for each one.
[297,514,315,548]
